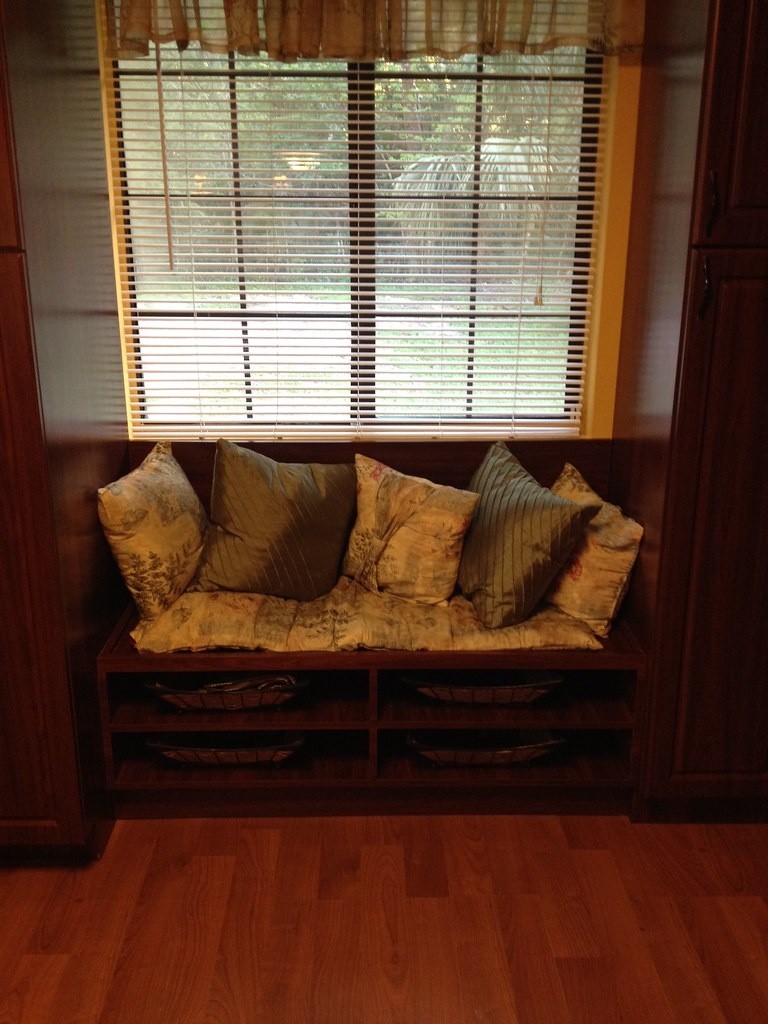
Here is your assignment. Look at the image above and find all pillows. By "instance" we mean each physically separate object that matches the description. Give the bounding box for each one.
[540,465,640,639]
[98,437,206,618]
[465,437,594,626]
[345,454,469,601]
[189,443,351,606]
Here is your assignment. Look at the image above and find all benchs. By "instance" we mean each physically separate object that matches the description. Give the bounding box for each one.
[99,579,648,787]
